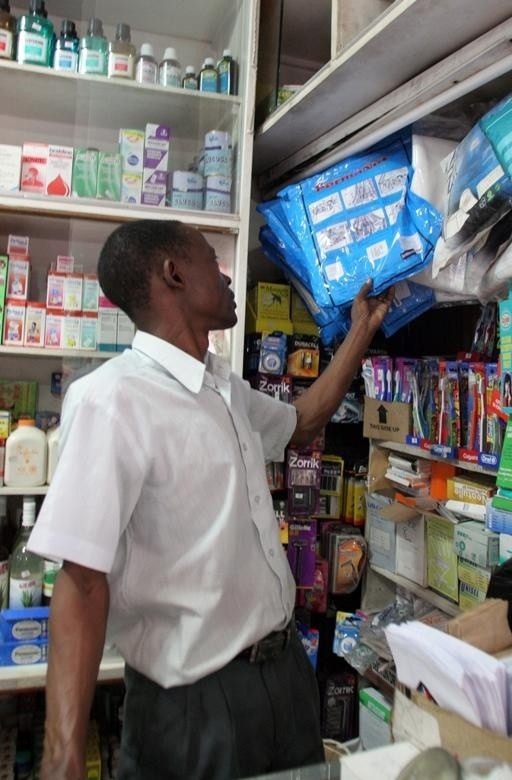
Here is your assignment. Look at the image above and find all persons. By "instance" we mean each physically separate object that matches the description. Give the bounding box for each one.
[24,219,396,779]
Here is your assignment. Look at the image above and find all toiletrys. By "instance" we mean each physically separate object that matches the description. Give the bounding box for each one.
[46,423,62,486]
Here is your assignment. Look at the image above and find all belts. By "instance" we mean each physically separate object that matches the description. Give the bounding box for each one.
[235,619,303,666]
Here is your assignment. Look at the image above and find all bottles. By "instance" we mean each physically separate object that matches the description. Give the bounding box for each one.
[0,0,238,95]
[2,497,58,609]
[47,423,60,483]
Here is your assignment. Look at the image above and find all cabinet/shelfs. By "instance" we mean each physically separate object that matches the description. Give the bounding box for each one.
[1,1,263,697]
[361,434,512,619]
